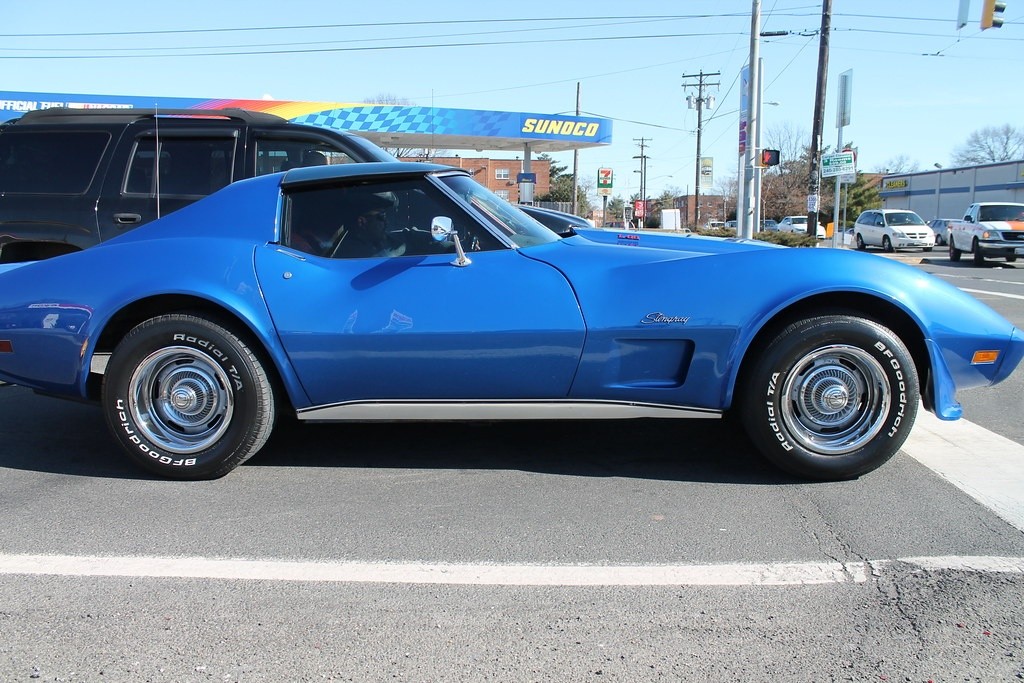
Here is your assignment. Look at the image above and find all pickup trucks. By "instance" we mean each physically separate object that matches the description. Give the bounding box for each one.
[945,201,1024,266]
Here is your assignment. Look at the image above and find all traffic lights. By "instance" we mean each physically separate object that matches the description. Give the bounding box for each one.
[761,148,781,166]
[981,0,1007,31]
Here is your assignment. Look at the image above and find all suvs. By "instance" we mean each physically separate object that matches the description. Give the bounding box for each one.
[0,108,594,264]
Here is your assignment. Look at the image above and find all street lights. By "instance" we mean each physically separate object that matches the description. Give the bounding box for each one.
[632,154,647,228]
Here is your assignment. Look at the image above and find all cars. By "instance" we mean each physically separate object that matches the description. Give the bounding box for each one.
[760,219,779,232]
[704,221,726,231]
[726,220,736,229]
[927,218,963,246]
[777,216,827,240]
[853,209,936,253]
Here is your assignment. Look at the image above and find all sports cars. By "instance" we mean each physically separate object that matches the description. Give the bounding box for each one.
[0,168,1024,480]
